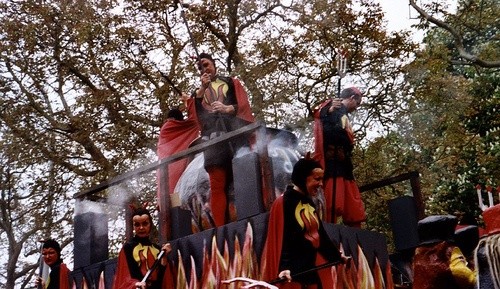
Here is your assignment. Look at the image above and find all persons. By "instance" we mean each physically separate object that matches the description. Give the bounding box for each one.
[113,206,174,289]
[270,157,351,289]
[35,238,73,289]
[314,87,367,229]
[191,52,257,226]
